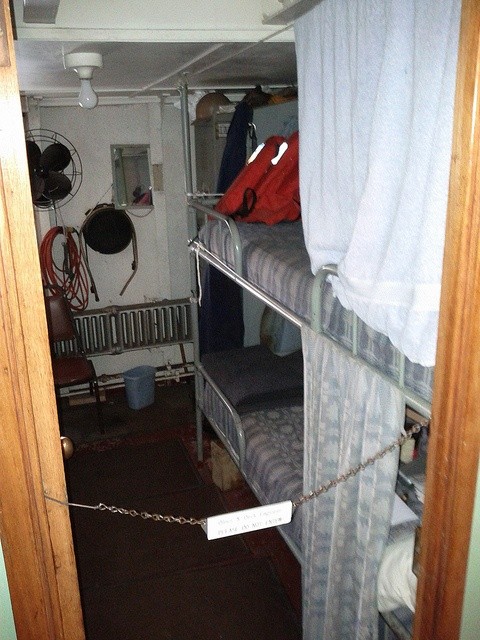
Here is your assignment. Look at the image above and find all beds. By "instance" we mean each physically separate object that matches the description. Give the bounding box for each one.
[197,343,429,640]
[192,190,440,417]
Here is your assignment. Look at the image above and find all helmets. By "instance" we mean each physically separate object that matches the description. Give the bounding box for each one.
[191,93,233,126]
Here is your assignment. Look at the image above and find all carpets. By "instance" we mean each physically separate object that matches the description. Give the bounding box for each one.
[63,434,199,505]
[72,486,253,592]
[79,554,304,640]
[57,383,204,443]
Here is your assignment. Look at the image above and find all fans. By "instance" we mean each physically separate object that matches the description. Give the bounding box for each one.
[25,126,82,215]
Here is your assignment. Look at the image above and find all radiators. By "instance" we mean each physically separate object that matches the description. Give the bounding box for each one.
[54,300,196,413]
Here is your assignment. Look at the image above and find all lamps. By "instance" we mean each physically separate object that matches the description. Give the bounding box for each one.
[63,52,102,110]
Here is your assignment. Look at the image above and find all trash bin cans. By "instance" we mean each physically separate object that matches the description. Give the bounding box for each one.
[123,366,157,410]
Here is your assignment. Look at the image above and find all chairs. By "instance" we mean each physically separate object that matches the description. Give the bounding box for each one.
[42,282,107,435]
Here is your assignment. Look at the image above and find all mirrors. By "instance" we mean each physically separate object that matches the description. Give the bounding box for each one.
[113,142,156,208]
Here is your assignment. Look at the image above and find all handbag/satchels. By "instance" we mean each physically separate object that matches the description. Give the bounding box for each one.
[209,130,300,226]
